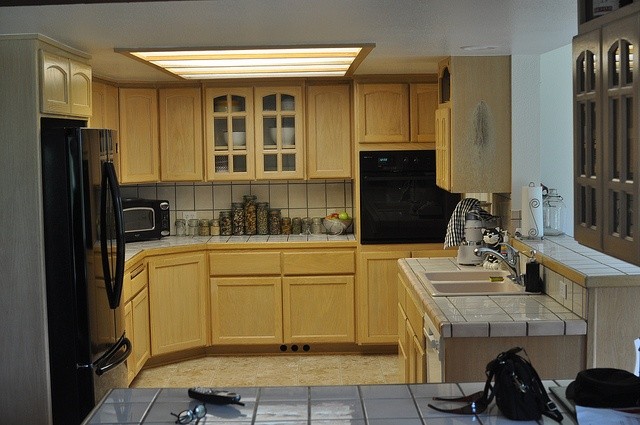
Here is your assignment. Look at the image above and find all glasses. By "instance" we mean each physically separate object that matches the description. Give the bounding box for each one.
[426,391,486,414]
[171,405,209,425]
[485,347,532,382]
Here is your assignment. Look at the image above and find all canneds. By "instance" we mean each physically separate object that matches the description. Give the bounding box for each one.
[199,218,208,236]
[210,219,220,235]
[187,219,198,235]
[313,216,322,235]
[282,217,290,235]
[175,218,185,236]
[292,217,299,234]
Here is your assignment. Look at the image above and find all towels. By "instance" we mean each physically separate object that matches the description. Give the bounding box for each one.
[444,197,498,250]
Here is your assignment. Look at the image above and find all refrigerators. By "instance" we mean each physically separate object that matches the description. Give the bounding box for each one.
[41,122,132,425]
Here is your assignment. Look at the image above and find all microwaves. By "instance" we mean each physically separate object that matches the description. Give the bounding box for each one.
[122,197,171,244]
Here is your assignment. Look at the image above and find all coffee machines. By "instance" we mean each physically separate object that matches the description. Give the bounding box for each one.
[457,199,501,265]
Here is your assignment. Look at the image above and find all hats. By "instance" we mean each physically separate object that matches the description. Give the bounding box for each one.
[565,367,639,405]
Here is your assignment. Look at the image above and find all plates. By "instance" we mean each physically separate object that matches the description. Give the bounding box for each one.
[215,156,229,172]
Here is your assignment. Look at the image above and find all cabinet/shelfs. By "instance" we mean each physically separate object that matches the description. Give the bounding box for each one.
[354,73,437,144]
[157,83,204,183]
[208,241,356,346]
[0,33,93,120]
[123,252,151,387]
[204,82,304,182]
[88,76,120,131]
[356,245,458,345]
[396,273,583,383]
[435,56,512,194]
[305,79,354,180]
[148,245,208,357]
[118,84,159,184]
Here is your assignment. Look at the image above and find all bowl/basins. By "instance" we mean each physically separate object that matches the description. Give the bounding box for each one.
[269,127,295,146]
[215,100,242,113]
[281,97,295,111]
[223,131,246,146]
[321,216,353,235]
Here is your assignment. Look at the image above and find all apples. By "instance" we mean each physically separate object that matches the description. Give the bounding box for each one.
[340,213,348,220]
[333,213,338,220]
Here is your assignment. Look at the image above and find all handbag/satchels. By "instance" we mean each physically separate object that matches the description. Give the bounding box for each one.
[481,356,564,422]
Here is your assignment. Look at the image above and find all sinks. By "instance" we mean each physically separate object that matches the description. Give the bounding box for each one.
[423,282,541,297]
[417,268,512,282]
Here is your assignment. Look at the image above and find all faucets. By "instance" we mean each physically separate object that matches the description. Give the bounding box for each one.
[473,242,525,287]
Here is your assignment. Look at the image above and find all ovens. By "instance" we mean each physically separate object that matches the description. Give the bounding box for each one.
[360,151,461,245]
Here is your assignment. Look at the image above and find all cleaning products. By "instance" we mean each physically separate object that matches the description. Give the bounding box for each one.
[501,231,511,271]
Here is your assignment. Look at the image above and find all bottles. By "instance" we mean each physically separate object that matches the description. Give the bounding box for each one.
[542,188,567,235]
[231,201,245,236]
[210,219,221,236]
[175,218,187,236]
[198,218,210,236]
[311,217,322,235]
[256,201,270,235]
[243,195,258,235]
[219,210,233,236]
[291,216,302,235]
[187,218,199,237]
[269,208,282,235]
[281,217,292,235]
